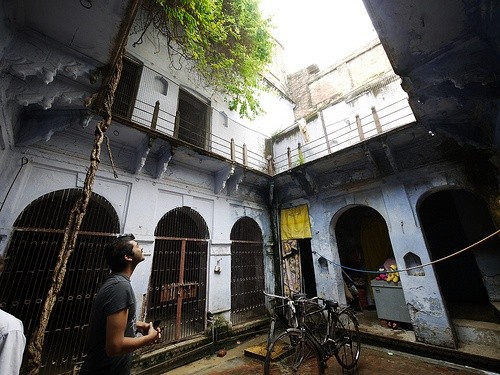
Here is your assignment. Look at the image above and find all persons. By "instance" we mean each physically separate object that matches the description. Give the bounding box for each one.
[77,234,161,375]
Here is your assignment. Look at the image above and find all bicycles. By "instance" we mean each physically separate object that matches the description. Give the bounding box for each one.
[261,289,362,375]
